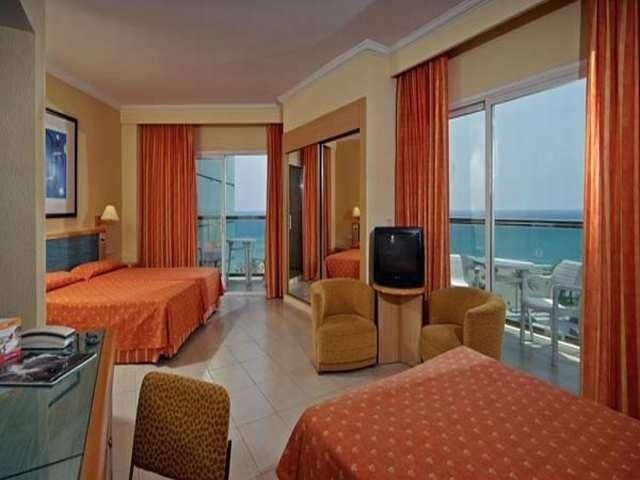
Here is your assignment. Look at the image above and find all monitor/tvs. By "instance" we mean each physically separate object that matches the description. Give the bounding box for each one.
[373,227,425,288]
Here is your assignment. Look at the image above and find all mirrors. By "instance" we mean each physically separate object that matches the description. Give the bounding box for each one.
[284,128,361,305]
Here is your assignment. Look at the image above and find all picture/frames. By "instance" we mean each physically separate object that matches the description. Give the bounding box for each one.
[46,106,78,219]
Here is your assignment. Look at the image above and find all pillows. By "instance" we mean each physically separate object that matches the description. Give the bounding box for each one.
[67,258,128,283]
[47,270,86,293]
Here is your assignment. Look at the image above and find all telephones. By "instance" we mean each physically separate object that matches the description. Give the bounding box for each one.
[20,325,76,350]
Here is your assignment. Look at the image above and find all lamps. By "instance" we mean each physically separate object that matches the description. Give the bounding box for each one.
[351,206,360,241]
[95,204,121,256]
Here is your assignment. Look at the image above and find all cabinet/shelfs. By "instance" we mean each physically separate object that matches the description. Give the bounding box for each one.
[0,325,119,480]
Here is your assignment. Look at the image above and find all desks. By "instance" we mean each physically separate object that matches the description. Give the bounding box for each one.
[372,282,427,368]
[226,239,254,288]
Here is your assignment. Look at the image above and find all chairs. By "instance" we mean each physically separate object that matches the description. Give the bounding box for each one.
[310,277,380,375]
[518,258,584,367]
[127,371,234,480]
[419,285,507,365]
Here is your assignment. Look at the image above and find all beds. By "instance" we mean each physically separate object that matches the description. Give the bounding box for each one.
[276,342,640,480]
[324,241,360,280]
[46,226,226,366]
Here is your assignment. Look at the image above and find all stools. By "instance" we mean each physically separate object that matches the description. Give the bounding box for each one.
[251,256,266,286]
[199,247,222,267]
